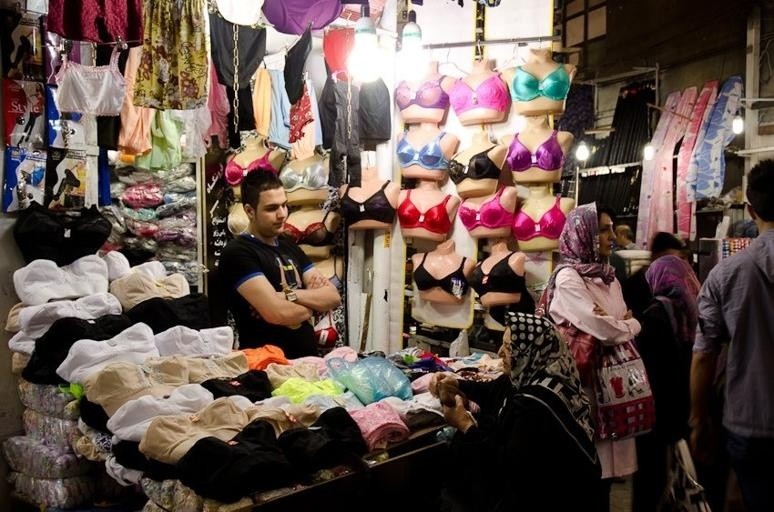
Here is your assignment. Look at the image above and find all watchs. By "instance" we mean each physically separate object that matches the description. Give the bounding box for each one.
[283,289,297,302]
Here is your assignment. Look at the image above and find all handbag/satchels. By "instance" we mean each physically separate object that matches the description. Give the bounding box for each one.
[534,308,596,387]
[591,342,655,441]
[654,465,711,511]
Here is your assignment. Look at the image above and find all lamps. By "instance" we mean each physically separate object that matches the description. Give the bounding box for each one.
[395,8,432,84]
[572,126,615,163]
[336,1,386,84]
[733,97,773,137]
[639,101,697,162]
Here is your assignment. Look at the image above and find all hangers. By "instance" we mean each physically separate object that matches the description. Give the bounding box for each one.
[491,44,526,71]
[435,43,471,77]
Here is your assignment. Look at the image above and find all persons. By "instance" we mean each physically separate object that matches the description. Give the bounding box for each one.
[336,167,400,233]
[684,156,773,509]
[217,166,343,357]
[397,179,461,242]
[409,239,476,304]
[280,203,341,246]
[621,230,683,312]
[609,226,633,252]
[504,113,575,185]
[633,256,702,497]
[222,135,287,201]
[396,123,461,182]
[219,202,255,238]
[16,151,45,208]
[510,183,576,253]
[276,146,330,190]
[303,309,340,353]
[446,60,509,126]
[540,200,644,511]
[428,312,604,510]
[467,242,526,308]
[505,42,577,117]
[447,130,511,195]
[7,27,37,77]
[297,255,344,292]
[48,159,85,209]
[396,59,455,125]
[456,184,518,239]
[16,83,44,144]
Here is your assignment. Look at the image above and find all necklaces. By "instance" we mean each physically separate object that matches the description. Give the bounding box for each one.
[250,234,274,246]
